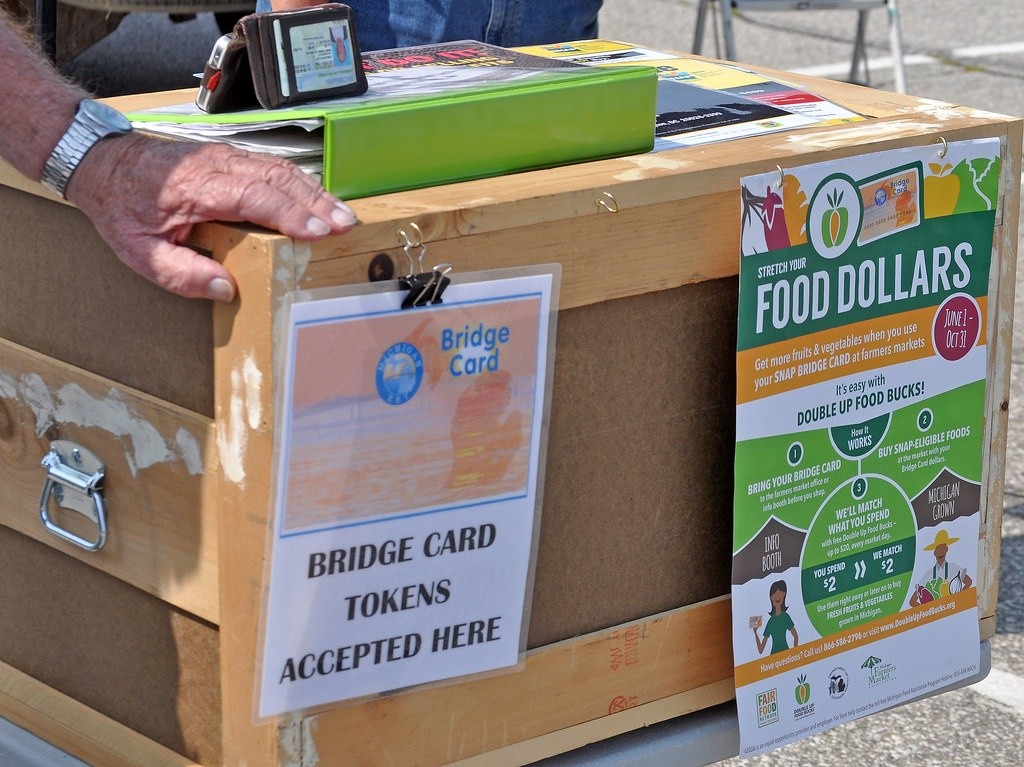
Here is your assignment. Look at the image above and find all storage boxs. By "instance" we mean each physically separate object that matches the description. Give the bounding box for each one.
[0,38,1024,767]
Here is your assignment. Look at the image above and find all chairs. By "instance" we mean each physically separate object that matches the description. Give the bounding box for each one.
[691,0,908,97]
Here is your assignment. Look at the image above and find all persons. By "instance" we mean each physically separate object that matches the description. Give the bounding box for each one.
[254,1,605,51]
[0,7,358,305]
[330,25,349,63]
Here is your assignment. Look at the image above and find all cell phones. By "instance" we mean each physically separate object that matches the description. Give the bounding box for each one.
[194,32,255,112]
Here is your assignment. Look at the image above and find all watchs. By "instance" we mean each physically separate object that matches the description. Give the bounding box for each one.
[39,98,133,201]
